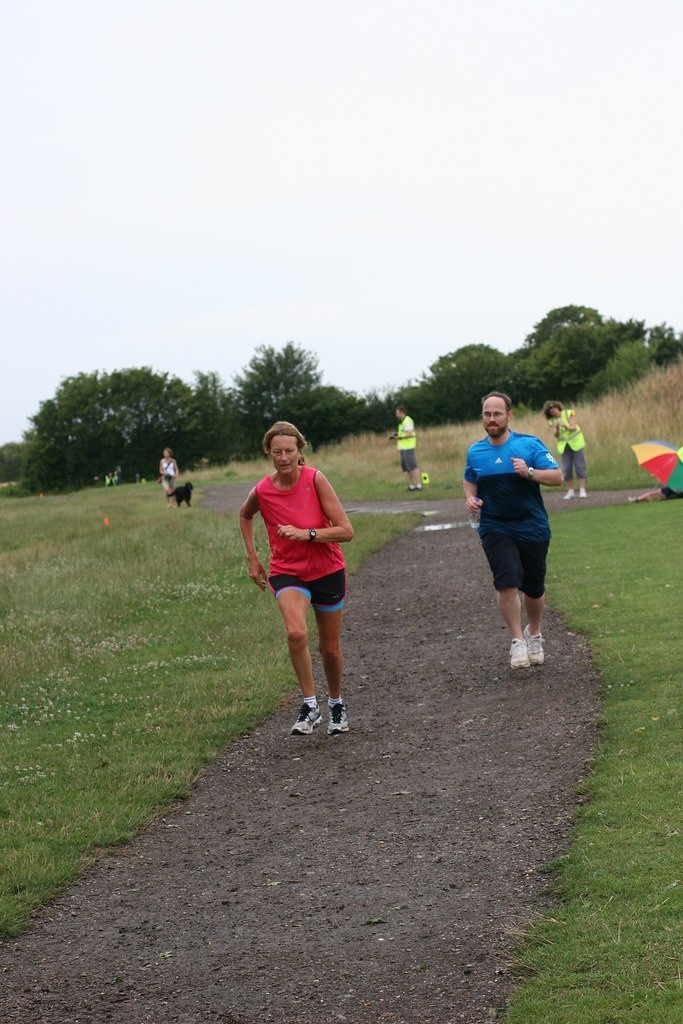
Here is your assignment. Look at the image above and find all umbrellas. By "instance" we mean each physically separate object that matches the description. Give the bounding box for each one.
[628,439,683,494]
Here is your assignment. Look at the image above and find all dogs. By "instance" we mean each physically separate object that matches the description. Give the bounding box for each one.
[166,482,193,508]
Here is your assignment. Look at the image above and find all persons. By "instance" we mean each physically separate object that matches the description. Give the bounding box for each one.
[238,421,355,736]
[111,470,121,486]
[104,471,114,486]
[159,448,179,509]
[462,391,565,669]
[627,485,682,504]
[542,399,588,501]
[389,406,423,491]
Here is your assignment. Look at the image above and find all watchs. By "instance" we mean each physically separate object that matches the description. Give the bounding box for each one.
[527,466,535,480]
[307,528,316,543]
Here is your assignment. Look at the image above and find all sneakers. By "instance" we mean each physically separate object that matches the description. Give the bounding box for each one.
[509,638,531,670]
[326,702,349,736]
[523,624,545,665]
[291,705,322,734]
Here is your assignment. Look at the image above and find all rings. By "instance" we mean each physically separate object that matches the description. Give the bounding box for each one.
[287,532,290,536]
